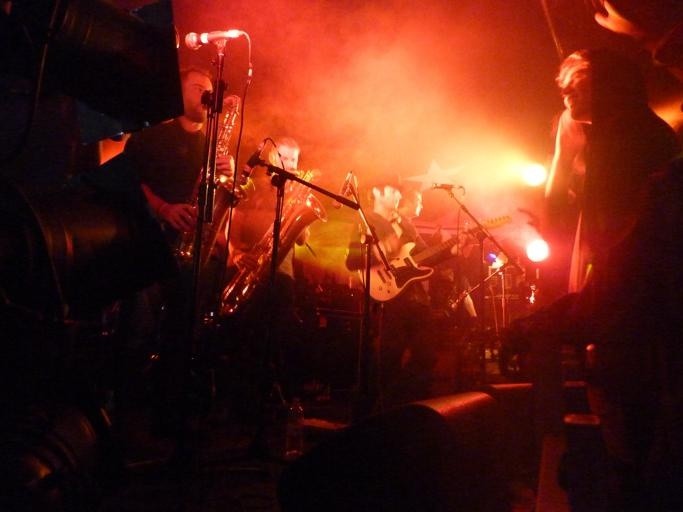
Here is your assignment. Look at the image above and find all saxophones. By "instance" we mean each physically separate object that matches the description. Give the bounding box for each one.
[169,94,248,275]
[217,170,329,317]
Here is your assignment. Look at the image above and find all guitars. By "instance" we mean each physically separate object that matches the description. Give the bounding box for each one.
[357,213,511,303]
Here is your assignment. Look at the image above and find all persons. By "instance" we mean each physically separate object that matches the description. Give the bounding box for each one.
[221,130,310,428]
[106,64,232,478]
[491,42,682,511]
[344,173,471,425]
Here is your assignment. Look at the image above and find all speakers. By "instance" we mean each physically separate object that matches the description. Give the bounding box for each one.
[479,374,595,476]
[338,391,517,511]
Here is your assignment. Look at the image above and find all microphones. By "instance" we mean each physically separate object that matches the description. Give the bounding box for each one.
[239,141,267,186]
[429,182,460,190]
[333,172,352,210]
[185,29,241,51]
